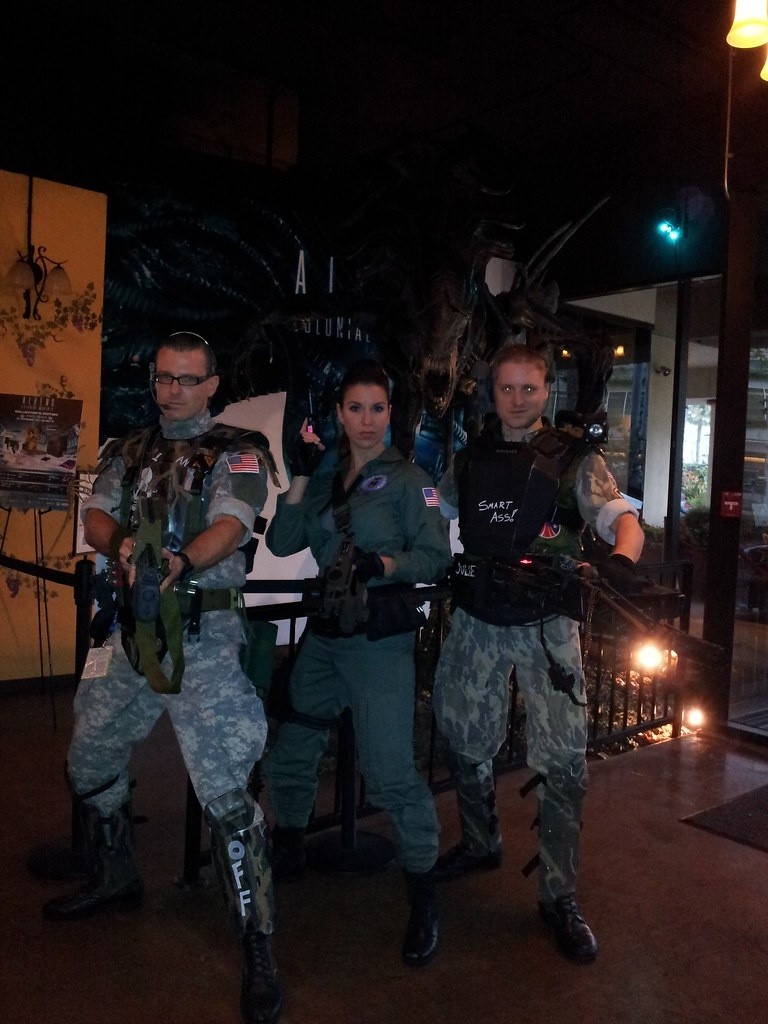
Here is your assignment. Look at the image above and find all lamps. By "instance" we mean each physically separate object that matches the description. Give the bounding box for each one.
[4,246,72,320]
[656,366,671,376]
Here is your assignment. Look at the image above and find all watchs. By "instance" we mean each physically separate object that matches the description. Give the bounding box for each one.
[174,552,194,582]
[361,551,384,580]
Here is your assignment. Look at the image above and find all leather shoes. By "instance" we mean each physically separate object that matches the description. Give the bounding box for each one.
[435,839,502,883]
[42,878,142,921]
[538,895,599,961]
[239,933,282,1024]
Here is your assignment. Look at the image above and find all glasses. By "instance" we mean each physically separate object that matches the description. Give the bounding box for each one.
[153,373,217,386]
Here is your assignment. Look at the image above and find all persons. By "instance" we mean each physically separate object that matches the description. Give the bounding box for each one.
[434,343,645,966]
[45,332,285,1024]
[266,371,452,965]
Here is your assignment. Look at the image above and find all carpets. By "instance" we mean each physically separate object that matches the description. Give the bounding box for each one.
[679,782,767,851]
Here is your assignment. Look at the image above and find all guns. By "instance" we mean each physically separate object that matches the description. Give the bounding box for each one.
[126,497,168,623]
[304,384,328,472]
[493,552,731,668]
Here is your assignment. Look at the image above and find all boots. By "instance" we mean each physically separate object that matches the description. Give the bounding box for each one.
[267,823,306,883]
[400,864,442,967]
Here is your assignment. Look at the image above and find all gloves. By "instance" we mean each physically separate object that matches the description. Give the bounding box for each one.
[597,554,639,597]
